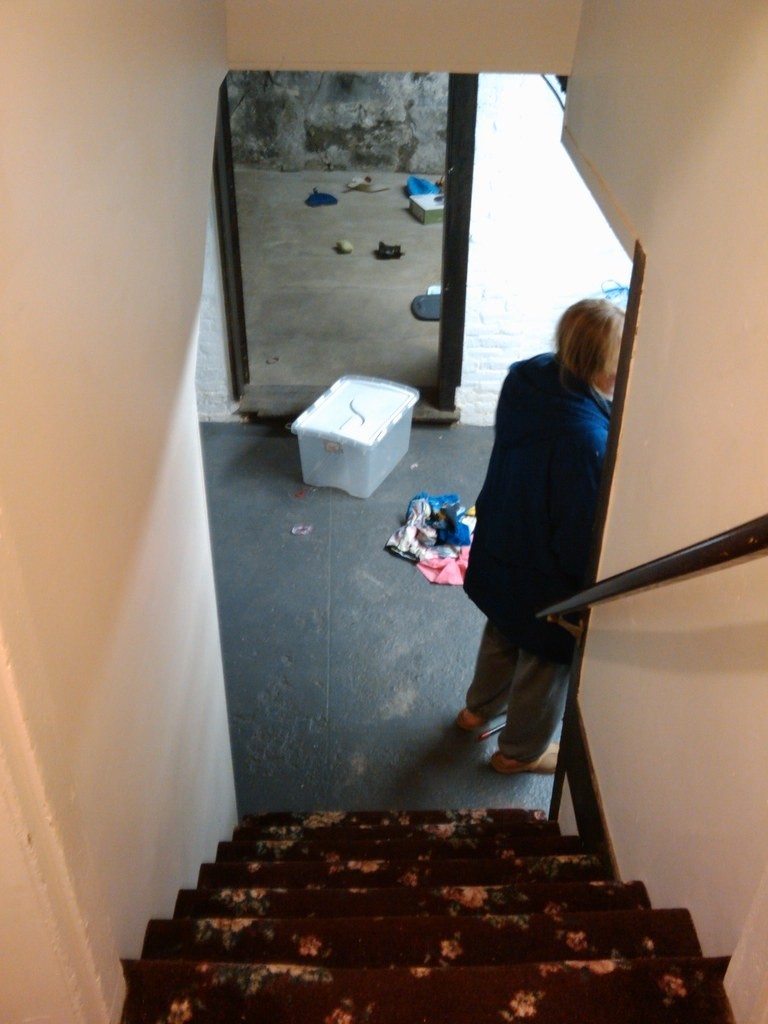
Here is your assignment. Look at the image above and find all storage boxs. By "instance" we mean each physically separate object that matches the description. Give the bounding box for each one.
[290,373,420,500]
[409,193,444,225]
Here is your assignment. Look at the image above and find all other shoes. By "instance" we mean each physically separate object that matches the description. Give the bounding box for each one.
[492,742,560,774]
[456,701,509,729]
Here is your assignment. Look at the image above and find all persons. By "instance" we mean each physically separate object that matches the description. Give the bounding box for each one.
[459,295,625,775]
